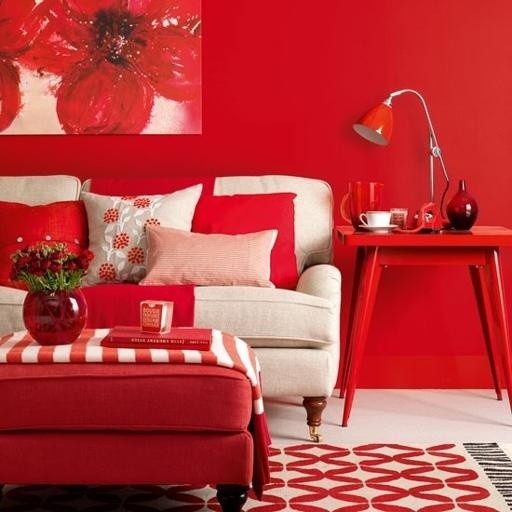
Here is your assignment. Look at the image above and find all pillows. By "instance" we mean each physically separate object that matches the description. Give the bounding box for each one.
[3,200,87,290]
[81,183,202,288]
[197,193,299,288]
[144,225,277,290]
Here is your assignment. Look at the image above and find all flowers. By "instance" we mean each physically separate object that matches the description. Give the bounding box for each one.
[8,242,95,292]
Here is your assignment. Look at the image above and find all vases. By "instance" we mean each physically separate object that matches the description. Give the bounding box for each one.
[450,176,479,233]
[22,287,88,347]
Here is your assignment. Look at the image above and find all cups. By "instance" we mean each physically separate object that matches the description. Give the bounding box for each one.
[359,211,392,225]
[390,207,408,227]
[340,181,384,232]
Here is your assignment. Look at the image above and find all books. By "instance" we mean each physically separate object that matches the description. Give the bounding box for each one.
[100,326,214,352]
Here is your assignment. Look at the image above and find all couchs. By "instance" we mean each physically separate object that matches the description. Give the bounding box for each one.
[0,174,343,446]
[2,328,252,512]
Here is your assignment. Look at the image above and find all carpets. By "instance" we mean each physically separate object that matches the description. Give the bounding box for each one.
[0,442,512,512]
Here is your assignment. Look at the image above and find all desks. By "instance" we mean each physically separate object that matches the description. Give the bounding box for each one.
[340,226,512,427]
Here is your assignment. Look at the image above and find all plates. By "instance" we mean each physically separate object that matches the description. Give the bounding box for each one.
[358,225,399,234]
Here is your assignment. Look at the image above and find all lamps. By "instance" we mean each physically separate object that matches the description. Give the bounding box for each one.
[353,89,449,235]
